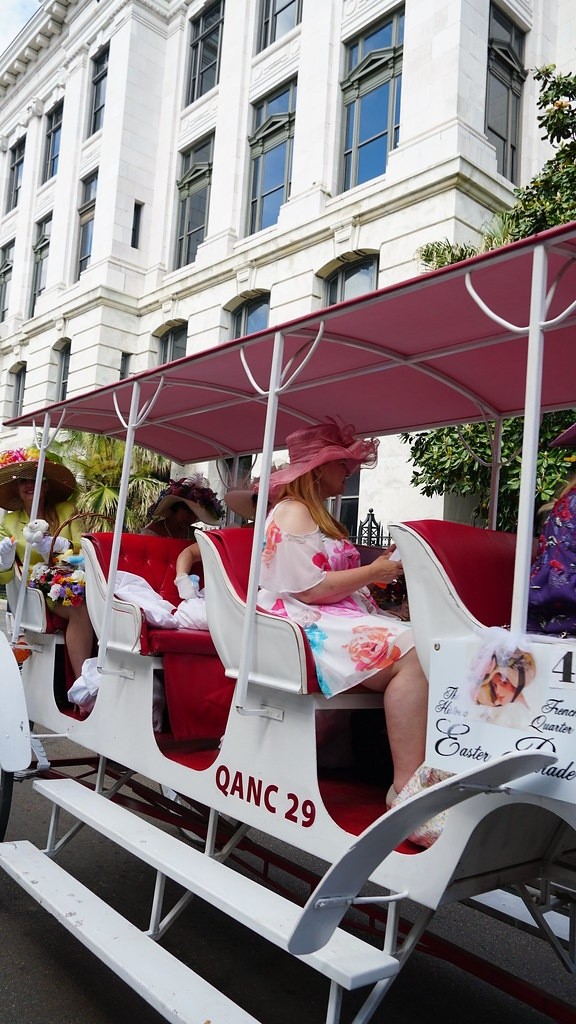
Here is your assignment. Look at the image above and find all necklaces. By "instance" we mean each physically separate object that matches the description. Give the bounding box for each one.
[164,521,189,539]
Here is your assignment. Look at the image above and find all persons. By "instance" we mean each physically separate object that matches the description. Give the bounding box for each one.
[139,473,226,539]
[474,648,536,730]
[253,421,429,811]
[528,421,576,636]
[0,447,98,716]
[173,464,289,600]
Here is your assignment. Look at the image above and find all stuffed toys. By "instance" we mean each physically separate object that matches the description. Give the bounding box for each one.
[23,519,50,543]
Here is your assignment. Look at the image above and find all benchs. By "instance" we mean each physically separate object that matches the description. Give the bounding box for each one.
[194,528,387,694]
[385,519,540,681]
[79,532,216,659]
[5,562,68,634]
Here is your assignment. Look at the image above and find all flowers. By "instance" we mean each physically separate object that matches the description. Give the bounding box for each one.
[147,477,225,521]
[0,447,40,469]
[28,551,87,608]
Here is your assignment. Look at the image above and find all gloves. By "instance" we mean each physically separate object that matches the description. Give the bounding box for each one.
[174,574,203,600]
[32,536,70,553]
[0,537,18,567]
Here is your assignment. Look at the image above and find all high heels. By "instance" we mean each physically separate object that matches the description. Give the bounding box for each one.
[386,784,398,811]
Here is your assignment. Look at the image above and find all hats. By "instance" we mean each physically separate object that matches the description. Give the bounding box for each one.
[548,422,576,447]
[146,477,226,526]
[253,416,380,494]
[0,448,76,511]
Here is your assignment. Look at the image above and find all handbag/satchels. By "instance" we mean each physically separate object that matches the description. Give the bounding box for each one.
[390,763,454,848]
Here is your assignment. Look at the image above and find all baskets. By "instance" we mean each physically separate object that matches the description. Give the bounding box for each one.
[45,513,130,606]
